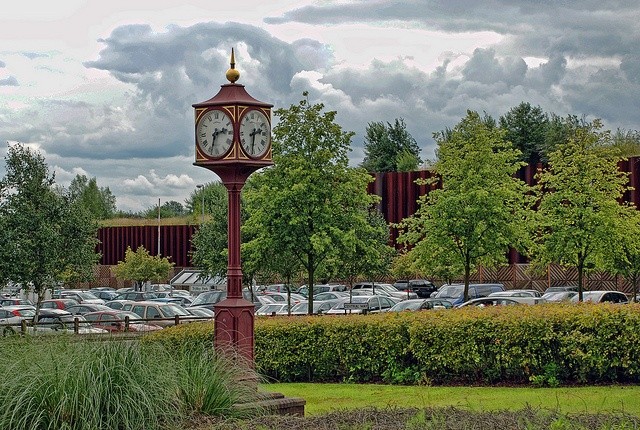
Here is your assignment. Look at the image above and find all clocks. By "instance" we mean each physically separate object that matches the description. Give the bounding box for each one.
[238,107,271,160]
[195,106,235,164]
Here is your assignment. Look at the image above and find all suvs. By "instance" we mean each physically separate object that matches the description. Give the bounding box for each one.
[31,313,108,333]
[121,302,207,326]
[265,284,298,293]
[348,283,419,300]
[393,280,437,298]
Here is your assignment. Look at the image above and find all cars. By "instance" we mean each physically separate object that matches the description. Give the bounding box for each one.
[388,298,452,312]
[290,301,336,315]
[58,290,105,305]
[489,293,532,297]
[1,299,37,307]
[1,305,36,336]
[106,301,134,311]
[314,290,350,300]
[83,311,163,332]
[90,284,226,306]
[244,286,263,295]
[454,297,542,307]
[253,304,293,317]
[508,288,540,297]
[542,292,576,303]
[64,304,113,315]
[326,296,396,315]
[185,307,215,318]
[41,298,79,309]
[268,294,309,305]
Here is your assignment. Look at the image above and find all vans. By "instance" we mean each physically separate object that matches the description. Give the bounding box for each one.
[570,290,629,304]
[430,284,506,305]
[544,286,585,293]
[298,285,346,294]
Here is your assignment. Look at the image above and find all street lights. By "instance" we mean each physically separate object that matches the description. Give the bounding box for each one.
[196,183,205,223]
